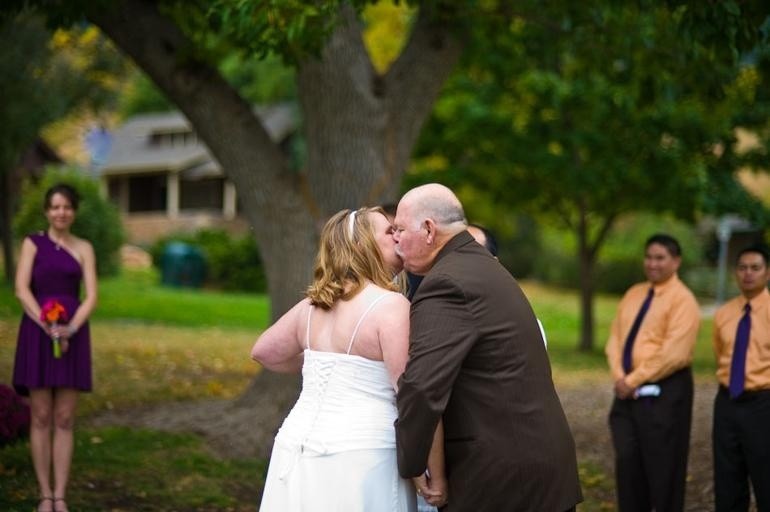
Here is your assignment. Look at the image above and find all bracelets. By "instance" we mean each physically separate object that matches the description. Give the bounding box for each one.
[66,323,77,337]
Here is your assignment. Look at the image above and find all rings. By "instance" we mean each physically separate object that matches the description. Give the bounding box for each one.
[416,488,422,495]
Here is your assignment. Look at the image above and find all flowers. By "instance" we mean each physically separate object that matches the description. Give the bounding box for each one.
[38,298,69,360]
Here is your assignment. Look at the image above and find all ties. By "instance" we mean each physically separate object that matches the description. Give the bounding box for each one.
[623,289,653,372]
[730,304,751,399]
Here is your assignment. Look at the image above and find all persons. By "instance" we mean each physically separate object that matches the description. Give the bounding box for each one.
[466,223,548,353]
[249,205,450,512]
[11,182,98,512]
[712,244,769,511]
[380,202,425,304]
[604,232,700,512]
[392,181,585,512]
